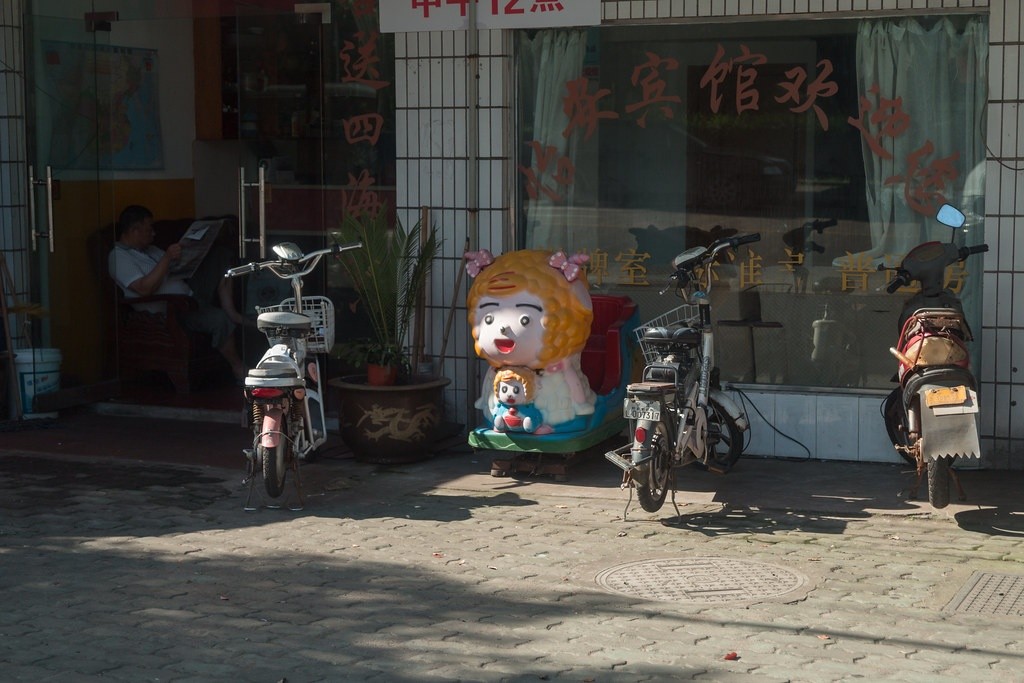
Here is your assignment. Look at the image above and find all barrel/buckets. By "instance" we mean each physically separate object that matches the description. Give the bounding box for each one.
[5,348,62,419]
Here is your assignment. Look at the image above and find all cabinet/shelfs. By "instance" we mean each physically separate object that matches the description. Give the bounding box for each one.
[192,1,324,141]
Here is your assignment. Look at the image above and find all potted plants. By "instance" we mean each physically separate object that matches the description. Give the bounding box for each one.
[326,205,450,458]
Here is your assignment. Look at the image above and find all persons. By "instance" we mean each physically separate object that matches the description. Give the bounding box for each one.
[108,204,292,390]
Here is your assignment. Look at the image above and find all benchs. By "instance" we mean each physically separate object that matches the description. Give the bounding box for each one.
[580,292,632,395]
[89,216,268,396]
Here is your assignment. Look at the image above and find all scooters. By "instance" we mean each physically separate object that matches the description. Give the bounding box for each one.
[224,231,362,511]
[877,202,989,509]
[604,232,761,525]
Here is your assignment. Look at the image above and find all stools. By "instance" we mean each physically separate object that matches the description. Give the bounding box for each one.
[717,320,783,384]
